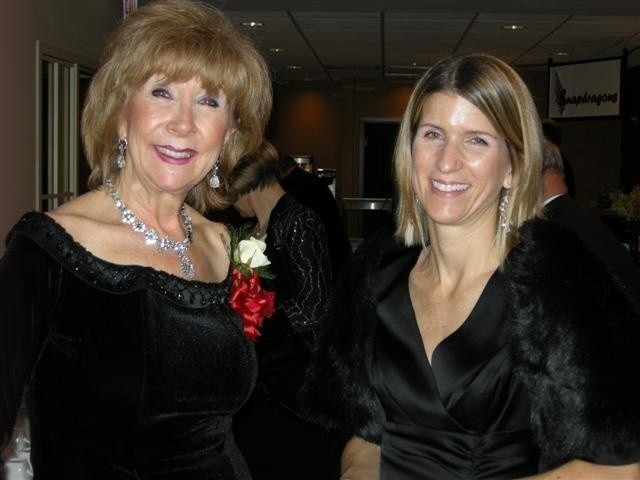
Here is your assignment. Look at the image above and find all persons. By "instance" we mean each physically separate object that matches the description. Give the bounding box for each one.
[275,151,353,270]
[540,117,579,198]
[521,133,640,300]
[284,53,640,480]
[0,0,273,480]
[221,139,334,479]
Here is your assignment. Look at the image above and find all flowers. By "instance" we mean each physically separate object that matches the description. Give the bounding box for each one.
[221,222,276,281]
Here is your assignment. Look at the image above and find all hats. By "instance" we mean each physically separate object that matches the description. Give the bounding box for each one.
[270,142,292,161]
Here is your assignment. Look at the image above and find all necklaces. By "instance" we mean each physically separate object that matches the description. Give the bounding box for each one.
[100,172,196,280]
